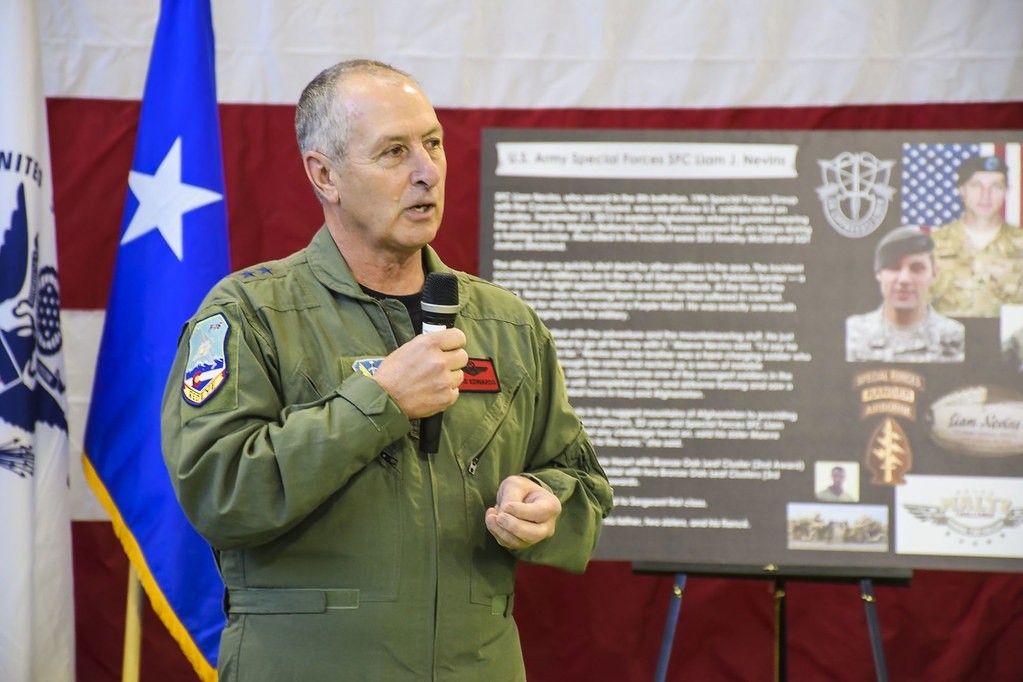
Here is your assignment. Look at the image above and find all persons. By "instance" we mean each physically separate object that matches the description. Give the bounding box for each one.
[819,466,855,504]
[930,156,1022,313]
[845,224,966,365]
[161,60,613,682]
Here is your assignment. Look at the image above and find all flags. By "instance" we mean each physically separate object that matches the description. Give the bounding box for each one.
[0,0,83,682]
[83,1,265,681]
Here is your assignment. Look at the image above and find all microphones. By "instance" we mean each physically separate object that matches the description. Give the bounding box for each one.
[418,271,461,453]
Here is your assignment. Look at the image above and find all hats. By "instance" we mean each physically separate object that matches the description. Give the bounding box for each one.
[960,156,1007,185]
[875,225,935,269]
